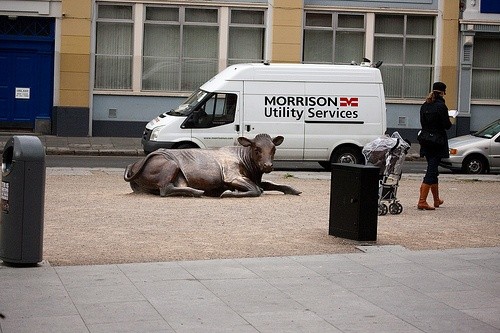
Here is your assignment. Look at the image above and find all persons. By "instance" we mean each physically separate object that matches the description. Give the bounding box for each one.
[417,82,452,211]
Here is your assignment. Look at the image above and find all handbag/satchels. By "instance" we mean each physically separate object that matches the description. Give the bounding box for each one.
[417,128,439,145]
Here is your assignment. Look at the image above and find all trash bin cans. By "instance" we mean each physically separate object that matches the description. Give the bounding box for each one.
[0,134,47,268]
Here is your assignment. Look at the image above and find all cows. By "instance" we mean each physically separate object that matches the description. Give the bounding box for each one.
[124,133,302,199]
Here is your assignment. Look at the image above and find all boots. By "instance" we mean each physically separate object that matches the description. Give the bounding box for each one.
[418,183,435,210]
[431,183,444,207]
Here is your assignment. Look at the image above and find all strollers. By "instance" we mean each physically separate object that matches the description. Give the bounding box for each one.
[363,131,412,215]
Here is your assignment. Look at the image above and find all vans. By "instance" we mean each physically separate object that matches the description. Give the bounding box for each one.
[140,55,389,171]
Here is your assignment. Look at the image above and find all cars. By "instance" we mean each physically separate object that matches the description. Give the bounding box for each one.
[440,123,500,174]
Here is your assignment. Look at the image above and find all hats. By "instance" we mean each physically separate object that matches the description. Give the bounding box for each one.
[433,82,446,95]
